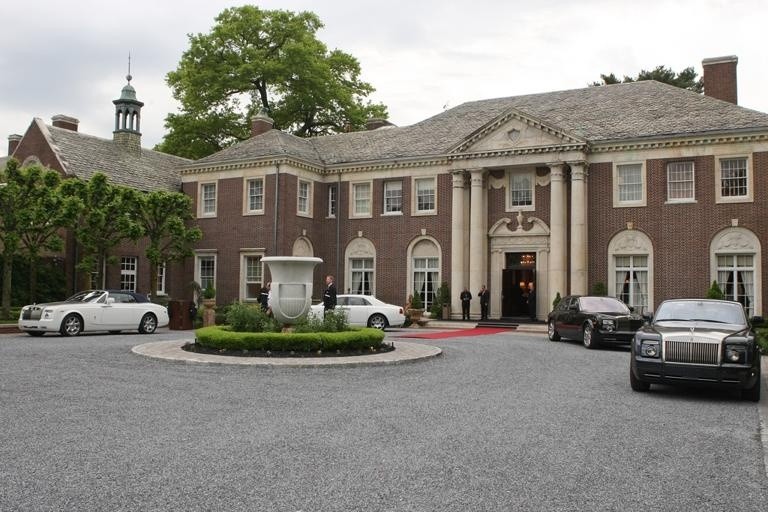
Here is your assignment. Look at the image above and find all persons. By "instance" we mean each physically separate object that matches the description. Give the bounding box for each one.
[258,280,268,314]
[459,286,473,320]
[322,275,336,321]
[527,281,535,319]
[478,284,490,320]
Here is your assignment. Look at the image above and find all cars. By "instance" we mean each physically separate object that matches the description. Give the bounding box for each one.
[306,294,406,331]
[548,295,647,349]
[630,297,760,402]
[18,289,170,337]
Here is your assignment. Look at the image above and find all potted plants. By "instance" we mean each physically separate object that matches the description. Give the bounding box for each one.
[202,280,215,327]
[408,291,425,329]
